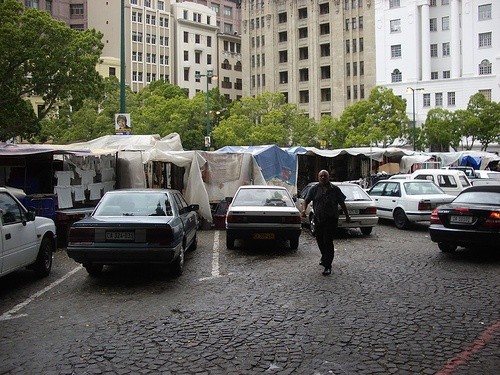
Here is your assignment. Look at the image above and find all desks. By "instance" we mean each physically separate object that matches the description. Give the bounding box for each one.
[54,207,94,223]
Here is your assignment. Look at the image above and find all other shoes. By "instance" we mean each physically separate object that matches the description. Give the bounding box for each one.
[324,268,330,275]
[319,258,323,265]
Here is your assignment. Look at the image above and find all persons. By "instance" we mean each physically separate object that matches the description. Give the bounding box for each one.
[117,115,129,129]
[302,170,350,275]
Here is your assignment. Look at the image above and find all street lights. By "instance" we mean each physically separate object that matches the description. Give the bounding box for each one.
[406,87,424,151]
[194,71,219,150]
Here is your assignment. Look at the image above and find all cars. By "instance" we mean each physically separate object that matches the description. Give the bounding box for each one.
[0,188,56,277]
[67,187,199,277]
[225,183,301,250]
[366,178,458,229]
[391,165,500,195]
[428,186,500,252]
[293,181,379,233]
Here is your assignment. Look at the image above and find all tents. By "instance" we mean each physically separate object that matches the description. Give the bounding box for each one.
[0,133,500,224]
